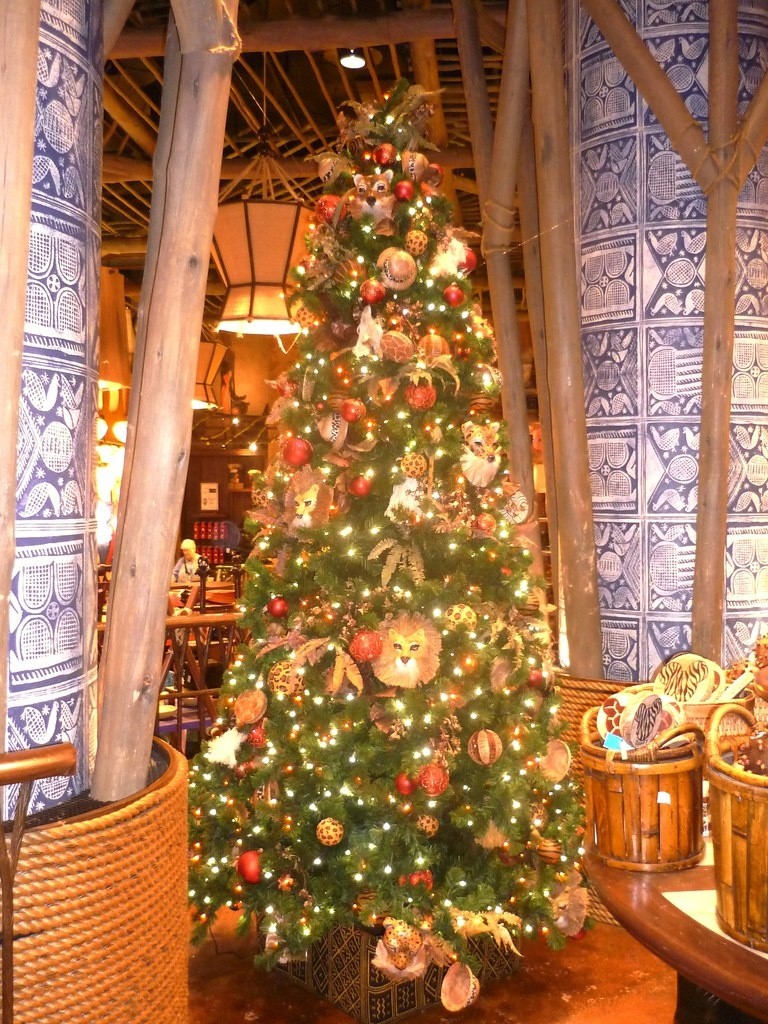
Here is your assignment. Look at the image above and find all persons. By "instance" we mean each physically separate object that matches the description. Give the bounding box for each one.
[173,539,210,600]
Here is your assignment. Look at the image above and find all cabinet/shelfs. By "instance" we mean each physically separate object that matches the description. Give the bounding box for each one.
[179,411,269,565]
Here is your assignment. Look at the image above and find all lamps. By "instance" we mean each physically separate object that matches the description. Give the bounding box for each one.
[99,266,131,388]
[193,341,228,409]
[210,53,316,334]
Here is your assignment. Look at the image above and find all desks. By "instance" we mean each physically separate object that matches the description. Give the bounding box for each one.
[584,780,768,1023]
[170,582,235,589]
